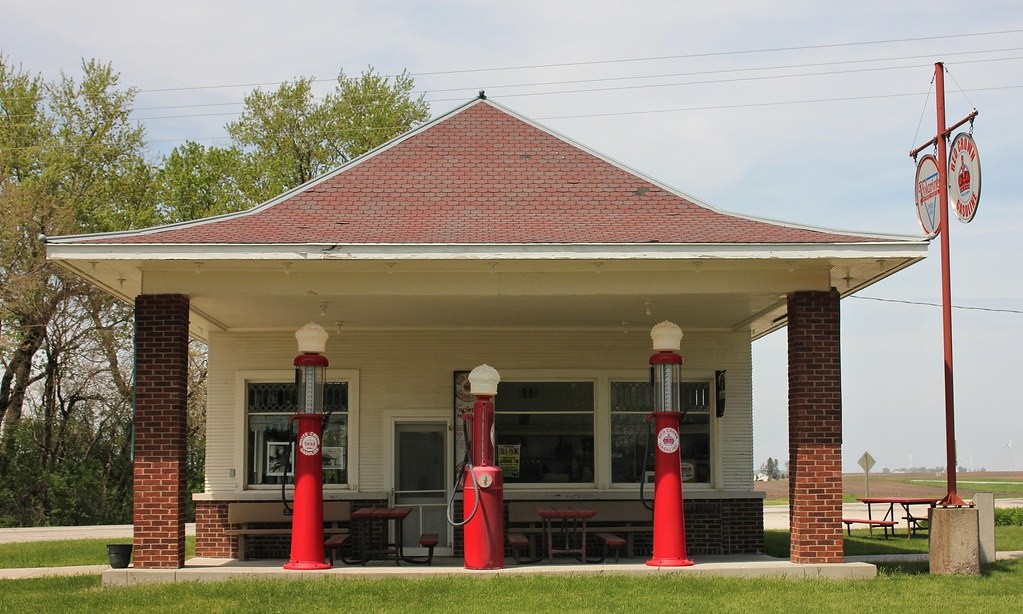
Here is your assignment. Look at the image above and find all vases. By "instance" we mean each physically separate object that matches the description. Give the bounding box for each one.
[106,544,133,569]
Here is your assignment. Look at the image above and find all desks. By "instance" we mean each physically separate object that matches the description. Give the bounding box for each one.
[857,498,943,539]
[513,508,607,565]
[341,507,433,565]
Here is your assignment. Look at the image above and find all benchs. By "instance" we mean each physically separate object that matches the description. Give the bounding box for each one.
[842,519,898,540]
[420,534,438,566]
[223,503,351,562]
[507,533,529,565]
[324,534,351,566]
[592,532,627,563]
[902,517,929,534]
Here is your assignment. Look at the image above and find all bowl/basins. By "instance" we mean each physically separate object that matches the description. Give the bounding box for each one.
[106,544,133,568]
